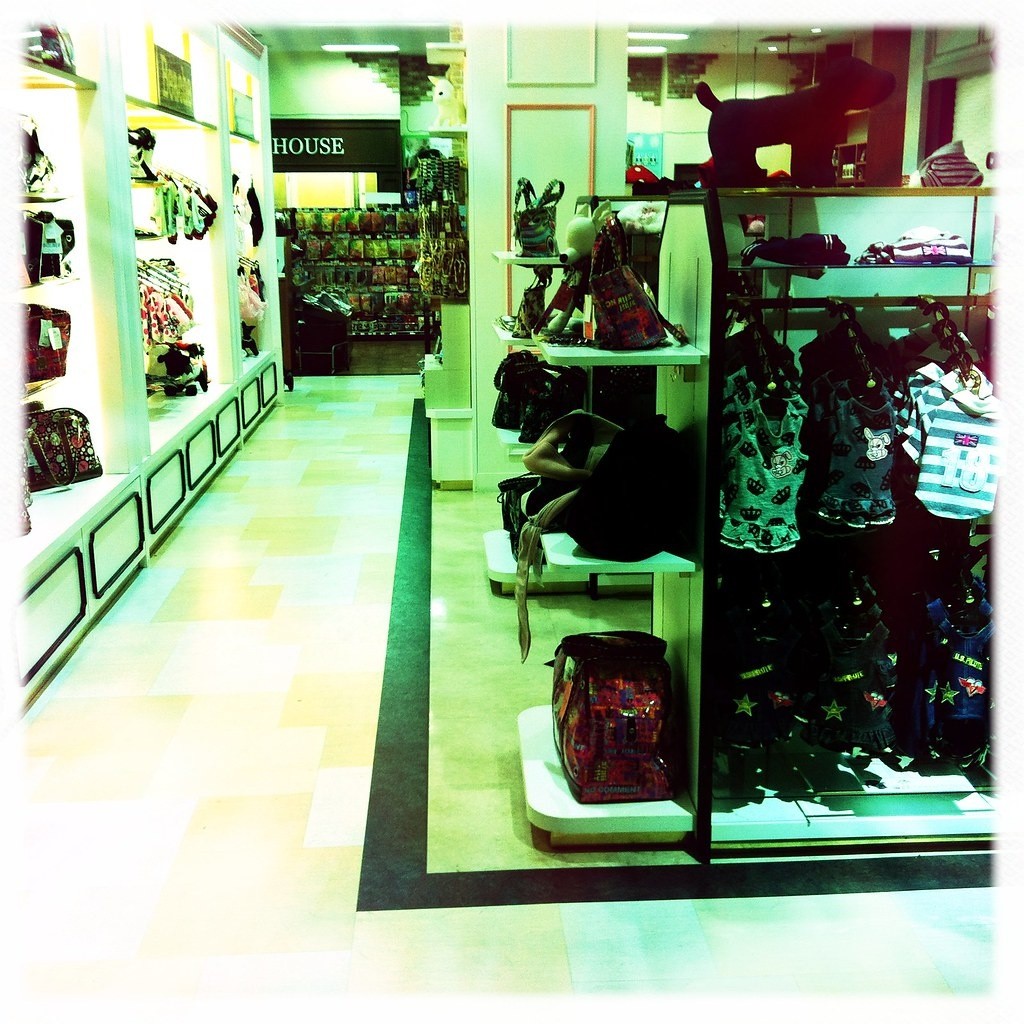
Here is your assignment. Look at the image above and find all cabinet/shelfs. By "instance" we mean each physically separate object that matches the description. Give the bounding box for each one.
[290,312,352,374]
[832,140,868,187]
[465,195,707,851]
[425,297,472,491]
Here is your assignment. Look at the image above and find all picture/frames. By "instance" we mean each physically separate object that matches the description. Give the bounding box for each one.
[506,23,599,87]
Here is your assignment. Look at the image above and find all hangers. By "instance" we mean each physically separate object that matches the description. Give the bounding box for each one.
[232,165,264,275]
[127,126,208,298]
[708,300,1002,436]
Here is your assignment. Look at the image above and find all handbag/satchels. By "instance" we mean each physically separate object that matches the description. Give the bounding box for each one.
[551,631,678,805]
[18,18,101,524]
[491,172,689,665]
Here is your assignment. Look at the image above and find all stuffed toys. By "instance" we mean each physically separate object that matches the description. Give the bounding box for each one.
[242,321,259,357]
[548,199,611,333]
[159,346,208,396]
[427,76,460,128]
[695,42,896,186]
[616,202,666,234]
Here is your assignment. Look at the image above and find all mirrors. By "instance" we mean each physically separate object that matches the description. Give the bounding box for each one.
[505,102,597,356]
